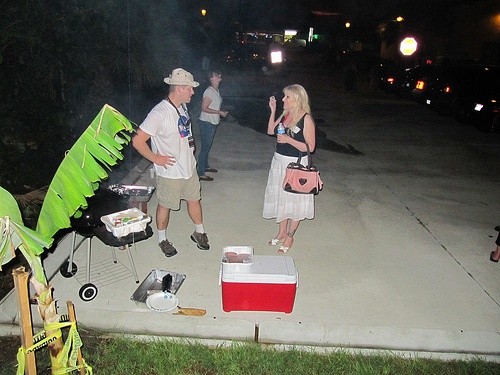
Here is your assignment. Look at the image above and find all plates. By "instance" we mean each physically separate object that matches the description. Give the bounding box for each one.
[147,293,178,312]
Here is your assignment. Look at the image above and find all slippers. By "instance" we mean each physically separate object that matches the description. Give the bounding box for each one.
[278,244,289,255]
[269,238,285,246]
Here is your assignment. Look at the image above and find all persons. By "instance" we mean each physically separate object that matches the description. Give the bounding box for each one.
[262,84,315,254]
[196,69,229,181]
[131,68,209,257]
[0,186,24,227]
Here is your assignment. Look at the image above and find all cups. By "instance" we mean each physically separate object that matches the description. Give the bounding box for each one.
[150,168,156,179]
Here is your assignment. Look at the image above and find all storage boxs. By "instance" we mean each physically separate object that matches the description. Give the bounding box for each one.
[219,254,299,314]
[221,245,254,271]
[99,206,151,238]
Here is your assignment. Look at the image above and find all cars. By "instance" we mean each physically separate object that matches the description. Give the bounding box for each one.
[220,42,270,97]
[374,36,500,135]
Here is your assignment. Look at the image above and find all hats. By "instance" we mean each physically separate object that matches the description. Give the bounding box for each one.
[163,68,200,88]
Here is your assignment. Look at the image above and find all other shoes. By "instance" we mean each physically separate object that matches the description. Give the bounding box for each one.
[199,175,213,181]
[490,251,499,262]
[204,168,217,173]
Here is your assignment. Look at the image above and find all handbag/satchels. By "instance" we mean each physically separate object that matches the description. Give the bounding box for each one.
[283,143,323,195]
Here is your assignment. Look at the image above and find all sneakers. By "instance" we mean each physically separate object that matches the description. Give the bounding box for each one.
[158,238,178,257]
[189,231,210,250]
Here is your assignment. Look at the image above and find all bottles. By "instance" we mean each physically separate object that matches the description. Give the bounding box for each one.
[278,123,285,135]
